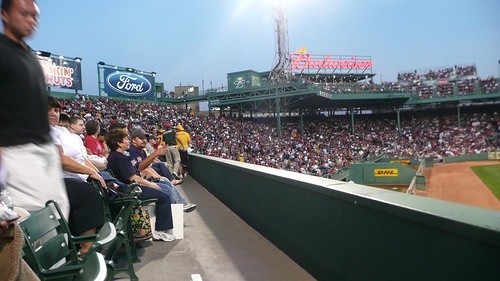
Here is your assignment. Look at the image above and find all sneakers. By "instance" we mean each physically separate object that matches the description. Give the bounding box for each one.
[183,203,196,213]
[153,230,176,242]
[173,179,184,185]
[172,172,181,178]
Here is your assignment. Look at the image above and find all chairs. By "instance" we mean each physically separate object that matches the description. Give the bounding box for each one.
[85,183,150,262]
[16,200,138,281]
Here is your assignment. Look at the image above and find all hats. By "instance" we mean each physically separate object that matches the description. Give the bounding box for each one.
[176,125,185,130]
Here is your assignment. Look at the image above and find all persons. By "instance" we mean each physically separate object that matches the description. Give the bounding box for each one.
[0,0,500,281]
[105,129,175,242]
